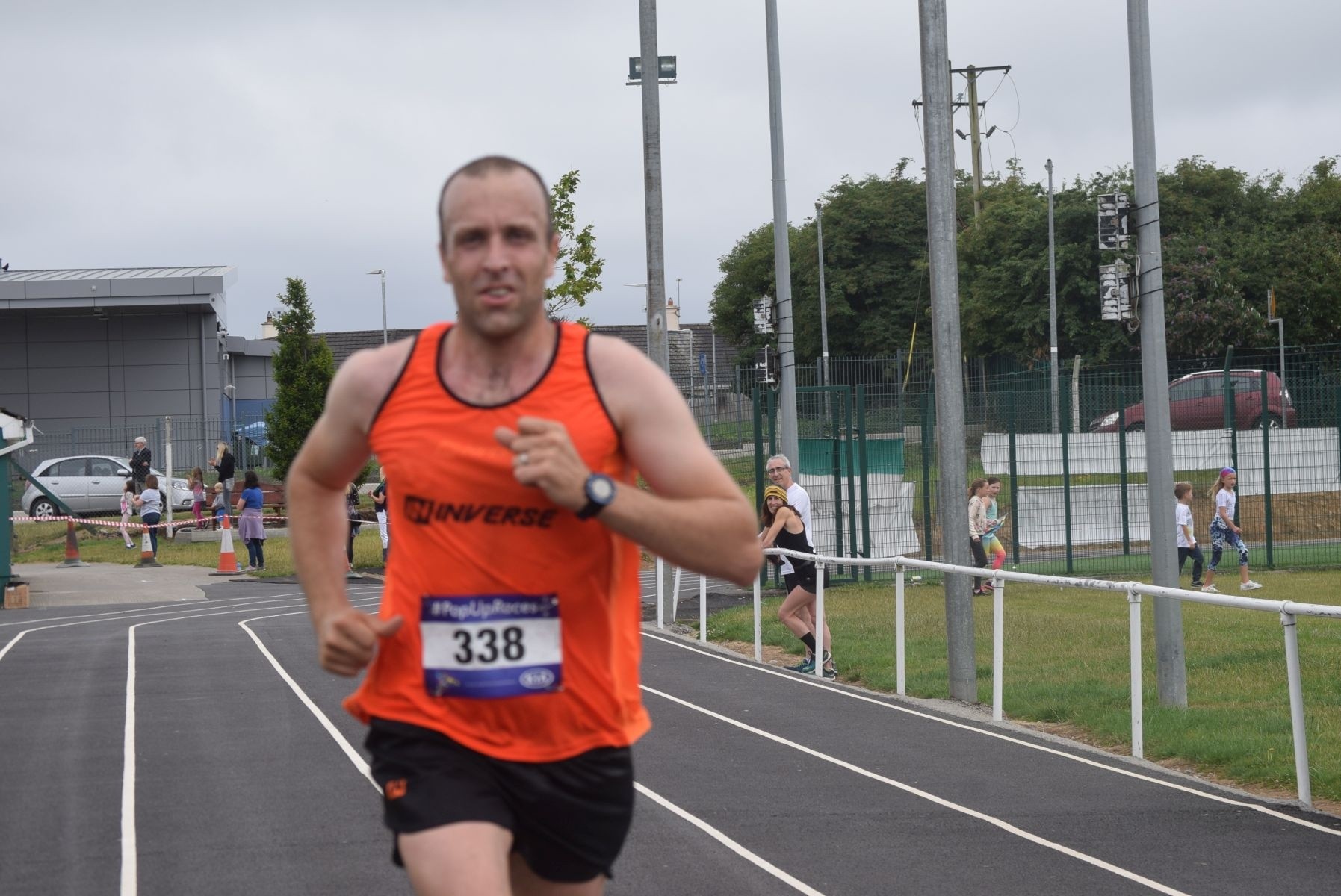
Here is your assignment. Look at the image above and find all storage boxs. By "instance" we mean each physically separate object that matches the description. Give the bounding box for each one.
[4,585,29,609]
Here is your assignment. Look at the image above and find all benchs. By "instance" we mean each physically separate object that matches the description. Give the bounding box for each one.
[206,482,286,531]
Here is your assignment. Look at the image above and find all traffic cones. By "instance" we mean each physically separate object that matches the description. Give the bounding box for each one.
[208,513,248,576]
[55,517,89,569]
[132,522,164,568]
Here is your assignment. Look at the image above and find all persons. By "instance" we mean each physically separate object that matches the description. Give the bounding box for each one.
[366,466,392,569]
[118,480,136,550]
[757,452,838,680]
[1172,483,1207,588]
[1200,467,1264,592]
[184,466,210,530]
[207,441,234,530]
[283,153,770,896]
[130,473,160,559]
[128,436,152,514]
[210,482,226,531]
[343,482,362,575]
[967,477,1010,596]
[235,470,267,572]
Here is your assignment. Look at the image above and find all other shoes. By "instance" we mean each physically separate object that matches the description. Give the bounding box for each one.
[204,521,209,528]
[126,546,131,550]
[131,543,136,548]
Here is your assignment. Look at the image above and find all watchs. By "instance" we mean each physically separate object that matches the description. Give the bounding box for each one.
[573,471,616,520]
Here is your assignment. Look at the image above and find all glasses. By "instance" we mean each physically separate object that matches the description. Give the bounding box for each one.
[767,467,787,475]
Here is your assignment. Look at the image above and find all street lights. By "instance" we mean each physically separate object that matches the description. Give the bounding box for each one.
[1268,317,1288,428]
[676,278,683,323]
[681,328,696,415]
[367,269,388,345]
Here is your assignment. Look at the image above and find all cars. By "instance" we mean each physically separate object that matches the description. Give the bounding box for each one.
[21,455,212,522]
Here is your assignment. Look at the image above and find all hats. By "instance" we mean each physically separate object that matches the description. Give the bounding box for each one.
[765,485,788,503]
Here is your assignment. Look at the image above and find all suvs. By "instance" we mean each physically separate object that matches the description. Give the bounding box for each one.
[1088,368,1299,433]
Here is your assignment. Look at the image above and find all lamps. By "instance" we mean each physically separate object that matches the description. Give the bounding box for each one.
[93,307,108,320]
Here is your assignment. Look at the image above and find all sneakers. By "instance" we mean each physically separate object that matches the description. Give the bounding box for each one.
[985,580,994,589]
[1241,580,1262,590]
[974,590,990,596]
[783,659,807,672]
[812,666,838,680]
[1190,581,1204,588]
[802,649,831,673]
[1202,584,1221,592]
